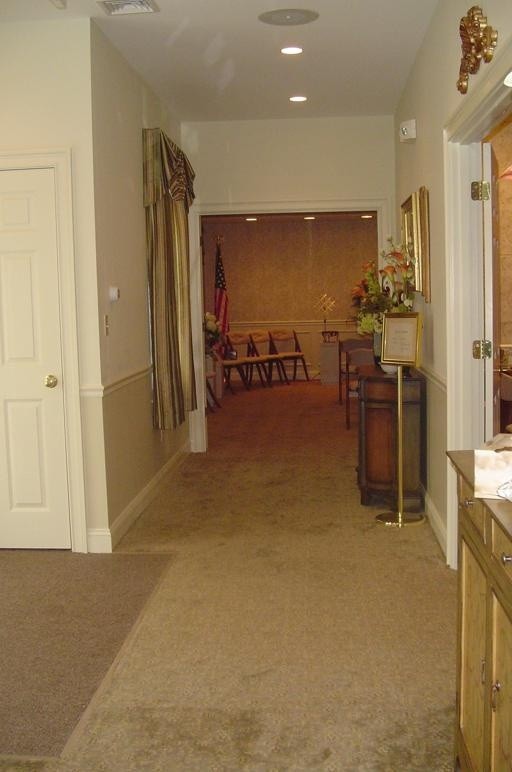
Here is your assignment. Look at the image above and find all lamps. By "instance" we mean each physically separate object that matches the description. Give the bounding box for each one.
[399,119,416,143]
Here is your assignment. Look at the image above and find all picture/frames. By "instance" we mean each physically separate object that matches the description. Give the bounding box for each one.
[401,193,418,291]
[419,185,431,304]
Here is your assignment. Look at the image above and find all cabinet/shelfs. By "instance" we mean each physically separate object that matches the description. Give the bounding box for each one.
[443,451,512,772]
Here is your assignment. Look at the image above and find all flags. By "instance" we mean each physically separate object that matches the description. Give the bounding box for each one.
[213,246,232,362]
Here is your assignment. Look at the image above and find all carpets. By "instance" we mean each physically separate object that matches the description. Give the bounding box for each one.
[0,550,177,763]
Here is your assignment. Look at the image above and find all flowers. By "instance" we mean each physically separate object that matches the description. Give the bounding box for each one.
[204,311,223,347]
[352,235,419,337]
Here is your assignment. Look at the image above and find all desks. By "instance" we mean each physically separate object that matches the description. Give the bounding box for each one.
[355,365,425,511]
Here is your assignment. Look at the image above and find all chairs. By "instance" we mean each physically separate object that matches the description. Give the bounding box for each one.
[338,338,377,406]
[221,329,310,394]
[345,350,395,430]
[206,371,223,414]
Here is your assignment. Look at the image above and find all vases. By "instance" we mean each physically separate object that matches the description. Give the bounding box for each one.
[203,343,214,357]
[373,331,383,356]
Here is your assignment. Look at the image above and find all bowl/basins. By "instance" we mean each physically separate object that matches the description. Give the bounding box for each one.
[379,363,399,375]
